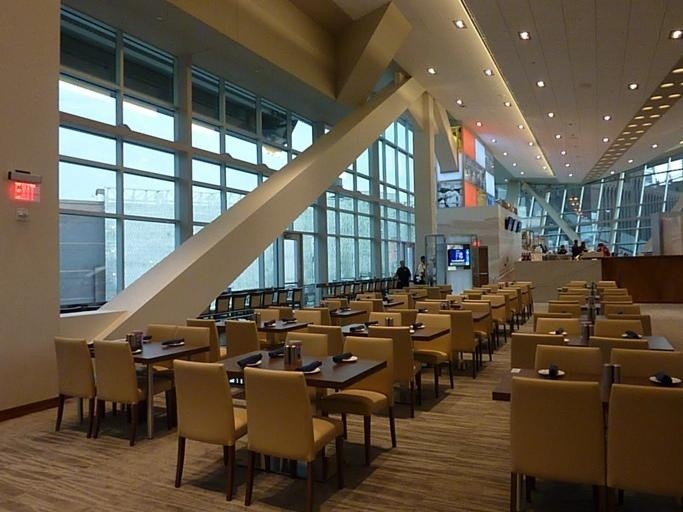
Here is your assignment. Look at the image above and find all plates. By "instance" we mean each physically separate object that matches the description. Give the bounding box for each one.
[373,323,381,326]
[538,368,565,375]
[267,324,276,327]
[548,331,567,335]
[141,338,153,342]
[416,308,428,313]
[167,341,185,346]
[299,367,320,374]
[343,355,358,362]
[343,308,352,311]
[246,360,262,366]
[356,329,364,331]
[649,375,681,384]
[276,353,285,357]
[288,319,298,323]
[622,334,642,339]
[409,325,425,329]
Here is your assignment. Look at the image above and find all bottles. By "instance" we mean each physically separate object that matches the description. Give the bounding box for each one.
[126,331,143,350]
[254,312,261,323]
[385,317,393,326]
[603,363,622,389]
[281,340,303,368]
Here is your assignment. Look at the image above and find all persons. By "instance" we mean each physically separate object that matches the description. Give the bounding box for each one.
[558,244,567,253]
[444,184,460,207]
[394,260,411,289]
[572,239,581,259]
[579,241,588,252]
[416,256,426,284]
[597,243,610,257]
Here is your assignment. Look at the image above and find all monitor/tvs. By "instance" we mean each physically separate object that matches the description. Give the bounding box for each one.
[516,221,522,233]
[505,216,513,231]
